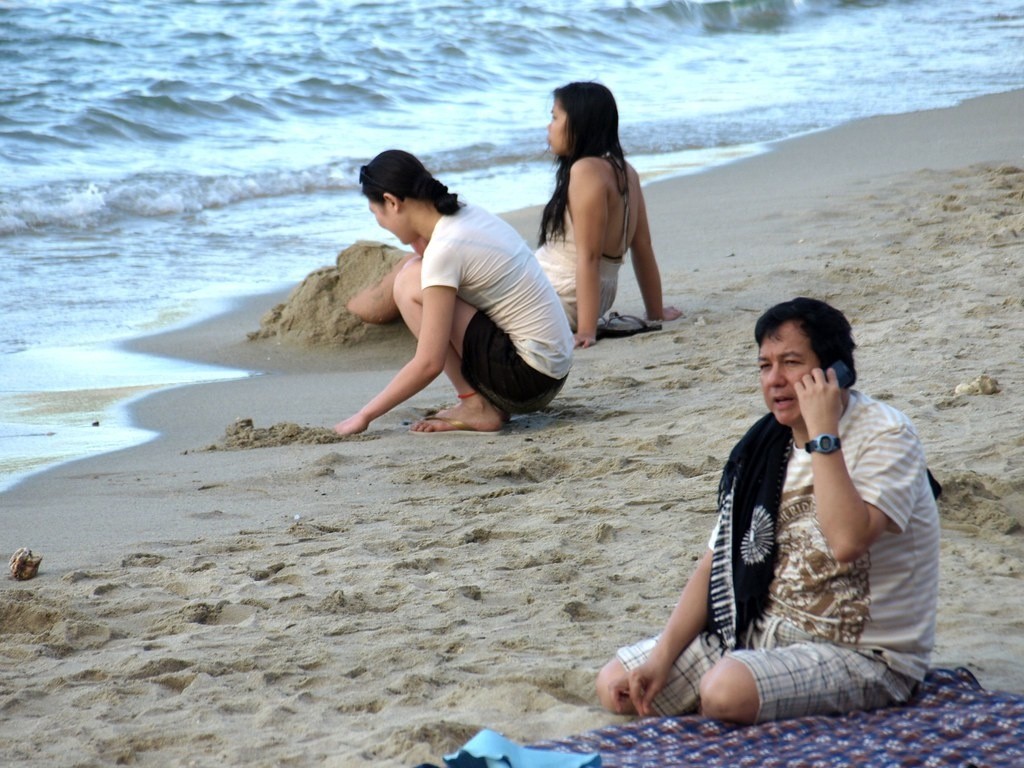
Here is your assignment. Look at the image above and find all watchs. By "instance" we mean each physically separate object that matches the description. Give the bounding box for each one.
[806,434,841,454]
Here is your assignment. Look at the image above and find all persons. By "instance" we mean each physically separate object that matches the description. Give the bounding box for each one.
[598,297,940,723]
[345,82,682,349]
[332,150,576,436]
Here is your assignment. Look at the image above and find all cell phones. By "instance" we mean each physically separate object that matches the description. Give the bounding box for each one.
[823,360,853,391]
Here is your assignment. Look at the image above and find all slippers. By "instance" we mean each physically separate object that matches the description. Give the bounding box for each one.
[603,312,662,338]
[595,316,607,341]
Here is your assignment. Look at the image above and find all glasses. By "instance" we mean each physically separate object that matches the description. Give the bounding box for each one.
[359,166,405,203]
